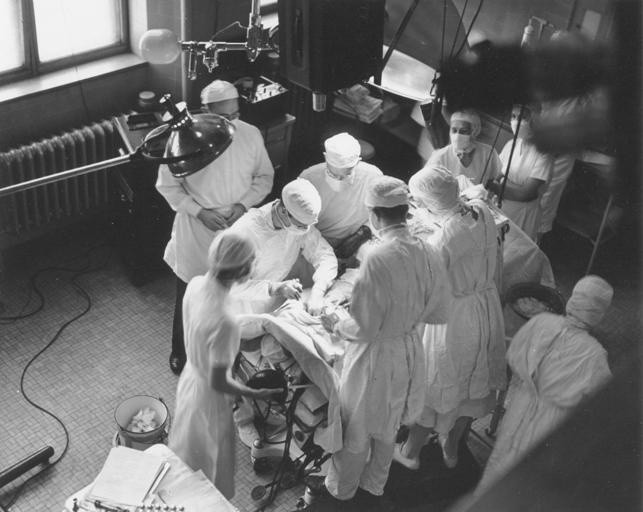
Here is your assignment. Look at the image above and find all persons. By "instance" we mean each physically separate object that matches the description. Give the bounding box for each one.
[489,98,553,249]
[533,90,589,250]
[390,165,511,474]
[471,273,614,497]
[425,106,501,193]
[287,133,386,292]
[224,175,339,450]
[165,228,283,500]
[152,79,276,378]
[303,175,455,507]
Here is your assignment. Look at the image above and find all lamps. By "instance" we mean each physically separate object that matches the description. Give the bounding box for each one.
[1,92,241,207]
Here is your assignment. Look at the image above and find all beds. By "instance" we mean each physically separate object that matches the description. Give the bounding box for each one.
[246,187,553,479]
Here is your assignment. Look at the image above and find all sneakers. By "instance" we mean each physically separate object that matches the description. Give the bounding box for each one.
[392,441,420,471]
[439,432,458,467]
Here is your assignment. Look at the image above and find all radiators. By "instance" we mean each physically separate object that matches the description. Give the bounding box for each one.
[0,116,120,244]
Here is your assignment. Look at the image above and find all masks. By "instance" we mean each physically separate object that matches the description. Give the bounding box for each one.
[367,212,409,239]
[324,167,356,192]
[449,133,471,151]
[274,205,310,238]
[510,120,532,139]
[222,264,254,284]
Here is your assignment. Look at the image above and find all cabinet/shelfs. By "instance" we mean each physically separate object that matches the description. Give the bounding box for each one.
[258,114,297,179]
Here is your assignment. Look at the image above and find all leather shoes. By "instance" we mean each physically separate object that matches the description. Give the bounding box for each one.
[169,351,186,373]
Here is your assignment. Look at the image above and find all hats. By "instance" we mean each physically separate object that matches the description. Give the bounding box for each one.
[408,164,460,214]
[567,274,614,328]
[364,175,409,208]
[324,132,361,169]
[282,178,322,225]
[200,79,240,104]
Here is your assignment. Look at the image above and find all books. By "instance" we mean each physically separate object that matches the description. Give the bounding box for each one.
[82,444,239,512]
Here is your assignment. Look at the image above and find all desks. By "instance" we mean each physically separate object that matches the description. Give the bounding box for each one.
[66,447,239,512]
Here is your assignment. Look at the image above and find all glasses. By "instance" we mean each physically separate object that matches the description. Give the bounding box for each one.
[220,112,240,120]
